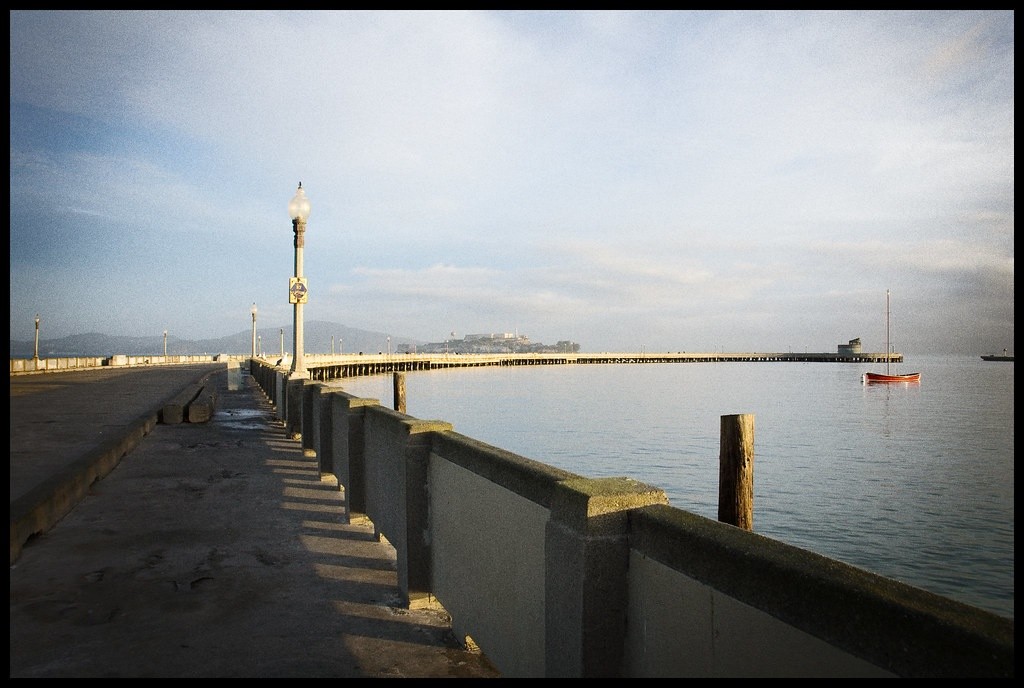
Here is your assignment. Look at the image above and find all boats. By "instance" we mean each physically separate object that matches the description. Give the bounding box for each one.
[980,348,1015,362]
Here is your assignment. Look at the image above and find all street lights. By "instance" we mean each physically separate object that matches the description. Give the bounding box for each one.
[339,339,343,355]
[257,334,262,358]
[34,313,41,359]
[250,302,258,358]
[386,336,391,354]
[288,179,312,379]
[163,330,167,356]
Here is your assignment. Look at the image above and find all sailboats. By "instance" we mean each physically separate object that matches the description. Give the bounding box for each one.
[861,289,922,383]
